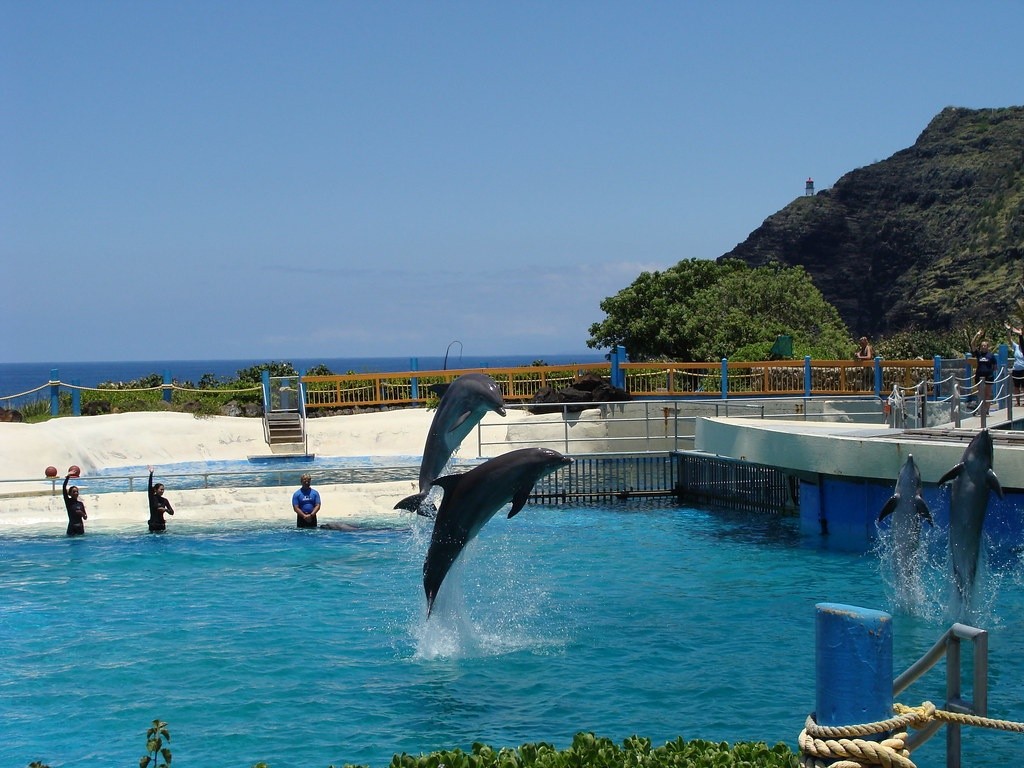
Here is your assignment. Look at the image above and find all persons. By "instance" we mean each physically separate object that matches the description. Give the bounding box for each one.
[292,473,321,528]
[854,337,874,391]
[146,464,174,531]
[970,329,997,416]
[62,470,87,536]
[1007,323,1024,407]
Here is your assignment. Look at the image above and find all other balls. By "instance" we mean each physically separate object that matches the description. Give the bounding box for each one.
[44,466,57,476]
[68,466,81,476]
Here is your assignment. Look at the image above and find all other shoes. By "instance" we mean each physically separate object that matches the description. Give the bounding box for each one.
[1015,402,1020,407]
[986,410,989,417]
[1021,403,1024,407]
[975,413,982,416]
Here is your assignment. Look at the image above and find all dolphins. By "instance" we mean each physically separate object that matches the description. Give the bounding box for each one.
[394,372,506,518]
[938,429,1003,609]
[424,447,575,619]
[879,453,934,578]
